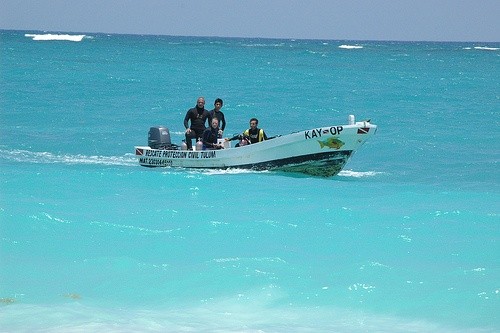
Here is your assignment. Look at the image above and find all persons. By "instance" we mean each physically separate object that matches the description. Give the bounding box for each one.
[184,97,226,150]
[224,118,276,147]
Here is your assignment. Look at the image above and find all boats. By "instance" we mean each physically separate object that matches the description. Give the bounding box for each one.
[474,44,499,50]
[134,115,377,178]
[33,33,85,42]
[339,44,363,50]
